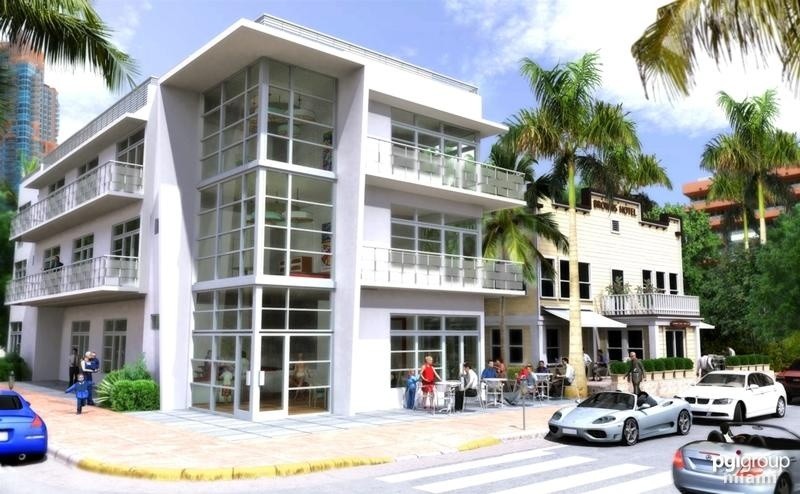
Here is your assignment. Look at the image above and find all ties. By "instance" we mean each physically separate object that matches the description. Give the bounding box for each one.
[73,356,77,366]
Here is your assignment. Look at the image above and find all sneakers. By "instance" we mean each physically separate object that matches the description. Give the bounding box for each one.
[504,396,517,405]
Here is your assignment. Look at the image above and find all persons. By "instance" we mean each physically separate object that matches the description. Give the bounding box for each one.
[481,345,607,406]
[240,350,249,400]
[81,350,96,405]
[404,367,422,410]
[220,365,235,402]
[63,372,96,415]
[66,347,79,389]
[455,360,477,412]
[420,355,444,409]
[626,352,645,398]
[293,351,311,402]
